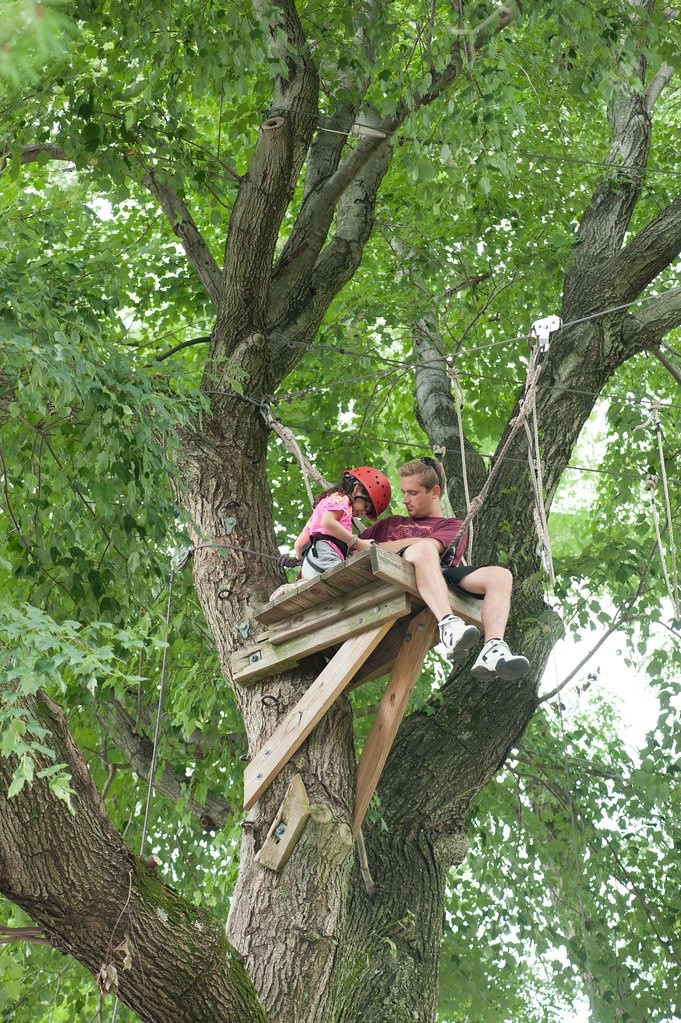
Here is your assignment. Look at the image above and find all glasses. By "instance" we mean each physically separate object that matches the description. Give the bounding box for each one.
[420,456,441,488]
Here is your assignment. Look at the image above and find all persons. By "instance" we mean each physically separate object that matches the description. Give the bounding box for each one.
[351,457,530,681]
[269,466,391,601]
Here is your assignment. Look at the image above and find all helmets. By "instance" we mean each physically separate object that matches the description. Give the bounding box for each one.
[343,466,392,522]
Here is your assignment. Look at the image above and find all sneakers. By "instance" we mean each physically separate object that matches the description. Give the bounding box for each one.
[439,614,479,660]
[469,639,530,683]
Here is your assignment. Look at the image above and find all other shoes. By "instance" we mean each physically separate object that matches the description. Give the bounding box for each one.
[269,577,309,603]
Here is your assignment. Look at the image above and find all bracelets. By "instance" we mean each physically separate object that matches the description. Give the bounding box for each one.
[347,536,356,548]
